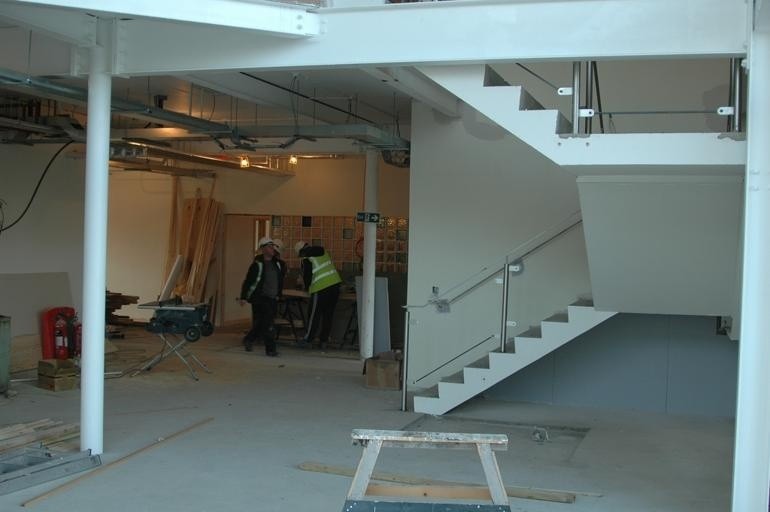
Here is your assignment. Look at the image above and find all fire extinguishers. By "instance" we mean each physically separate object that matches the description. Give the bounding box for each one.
[55,312,82,360]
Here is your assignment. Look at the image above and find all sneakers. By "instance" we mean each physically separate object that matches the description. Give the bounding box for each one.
[265,351,281,357]
[242,336,253,352]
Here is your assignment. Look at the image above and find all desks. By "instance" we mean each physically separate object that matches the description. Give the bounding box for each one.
[127,300,213,383]
[273,288,357,351]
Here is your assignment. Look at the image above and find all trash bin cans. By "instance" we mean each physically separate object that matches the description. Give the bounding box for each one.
[0,315,17,399]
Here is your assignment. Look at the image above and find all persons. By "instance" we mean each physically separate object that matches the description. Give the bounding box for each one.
[237,237,286,356]
[294,240,343,349]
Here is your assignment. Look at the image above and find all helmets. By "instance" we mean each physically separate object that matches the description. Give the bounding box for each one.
[294,241,308,257]
[274,238,285,255]
[258,236,275,248]
